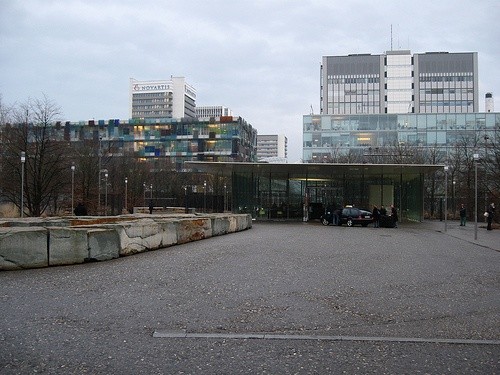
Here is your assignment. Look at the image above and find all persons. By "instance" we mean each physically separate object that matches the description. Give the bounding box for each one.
[344,201,349,208]
[149,200,153,214]
[272,202,277,208]
[74,203,88,216]
[459,202,466,226]
[372,205,398,228]
[484,201,496,230]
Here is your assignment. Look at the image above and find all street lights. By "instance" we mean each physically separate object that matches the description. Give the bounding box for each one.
[204,180,206,213]
[184,186,187,214]
[124,176,128,209]
[473,154,479,239]
[443,165,448,231]
[71,161,76,214]
[21,151,26,218]
[143,182,152,214]
[105,170,108,216]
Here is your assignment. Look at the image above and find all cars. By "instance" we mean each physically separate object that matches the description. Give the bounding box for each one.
[320,205,373,228]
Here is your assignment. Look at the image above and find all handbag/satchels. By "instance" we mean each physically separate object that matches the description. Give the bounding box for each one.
[483,211,489,217]
